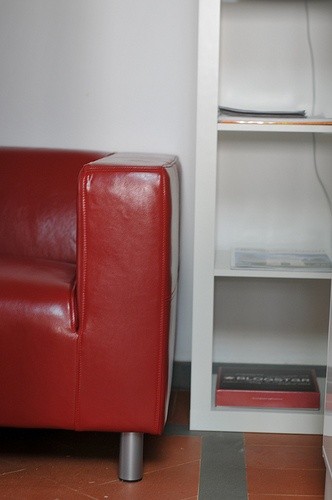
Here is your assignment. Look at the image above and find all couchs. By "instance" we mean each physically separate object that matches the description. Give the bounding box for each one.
[0,146,181,483]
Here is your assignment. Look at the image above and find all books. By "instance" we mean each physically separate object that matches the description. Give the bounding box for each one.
[219,107,308,117]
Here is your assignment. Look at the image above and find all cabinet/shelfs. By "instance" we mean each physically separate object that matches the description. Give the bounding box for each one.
[190,0,332,435]
